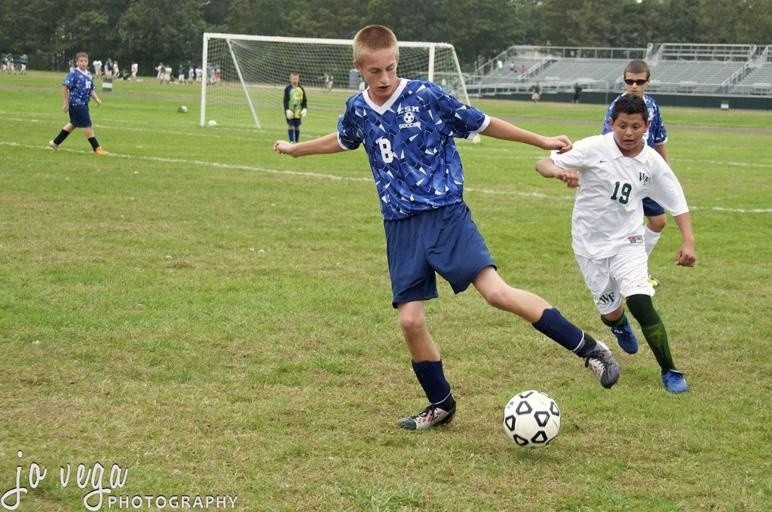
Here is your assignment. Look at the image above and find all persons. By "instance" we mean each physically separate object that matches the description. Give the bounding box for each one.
[112,59,119,79]
[131,60,139,81]
[69,57,76,73]
[154,62,166,85]
[326,72,334,90]
[187,59,198,81]
[164,64,172,85]
[206,63,214,86]
[272,24,621,429]
[534,93,697,394]
[48,51,111,155]
[283,71,308,145]
[104,57,114,80]
[509,60,514,71]
[602,59,672,289]
[571,82,582,104]
[213,61,221,85]
[530,82,540,104]
[519,65,526,82]
[194,65,202,82]
[2,52,12,72]
[19,52,29,74]
[178,64,186,84]
[496,59,504,71]
[6,55,17,76]
[92,57,103,79]
[318,71,329,89]
[116,66,132,81]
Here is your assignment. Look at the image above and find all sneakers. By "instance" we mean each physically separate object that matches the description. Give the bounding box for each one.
[396,402,456,430]
[611,321,644,355]
[93,145,110,159]
[582,339,624,390]
[48,139,61,151]
[646,272,660,288]
[662,369,689,394]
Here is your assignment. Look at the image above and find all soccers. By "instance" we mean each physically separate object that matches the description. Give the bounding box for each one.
[502,389,561,448]
[209,120,216,126]
[181,106,187,113]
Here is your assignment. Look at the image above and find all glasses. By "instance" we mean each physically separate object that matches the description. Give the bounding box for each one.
[625,79,647,86]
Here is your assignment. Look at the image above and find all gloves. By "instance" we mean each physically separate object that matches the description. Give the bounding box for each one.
[300,107,307,117]
[286,109,294,120]
